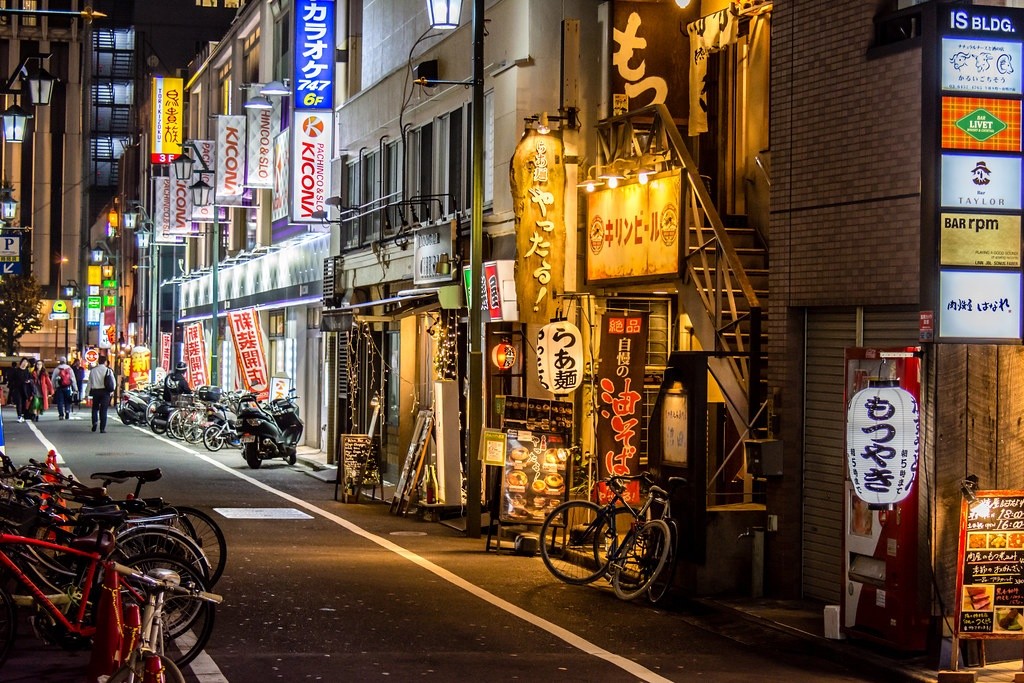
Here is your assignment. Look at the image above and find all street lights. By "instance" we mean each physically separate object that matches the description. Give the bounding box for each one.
[171,140,221,387]
[92,240,122,407]
[64,278,88,354]
[122,198,158,385]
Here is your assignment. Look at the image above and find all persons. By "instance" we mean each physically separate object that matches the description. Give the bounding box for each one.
[6,357,55,423]
[85,355,118,433]
[51,357,78,420]
[71,359,85,408]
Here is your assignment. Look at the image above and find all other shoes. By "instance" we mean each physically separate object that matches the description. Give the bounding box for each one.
[66,411,69,418]
[59,416,63,419]
[100,430,106,433]
[92,422,97,432]
[18,417,24,422]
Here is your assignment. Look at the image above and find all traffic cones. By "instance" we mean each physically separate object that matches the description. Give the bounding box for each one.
[85,564,168,683]
[39,448,73,540]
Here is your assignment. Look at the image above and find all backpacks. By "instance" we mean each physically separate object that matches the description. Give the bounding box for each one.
[58,366,72,386]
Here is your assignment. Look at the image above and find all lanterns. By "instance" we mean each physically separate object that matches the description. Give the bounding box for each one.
[846,379,920,511]
[536,315,584,398]
[492,343,516,370]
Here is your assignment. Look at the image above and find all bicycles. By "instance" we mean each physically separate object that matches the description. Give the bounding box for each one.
[539,471,681,604]
[166,385,267,452]
[0,451,227,667]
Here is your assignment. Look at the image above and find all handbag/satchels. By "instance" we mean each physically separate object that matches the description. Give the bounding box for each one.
[104,369,114,392]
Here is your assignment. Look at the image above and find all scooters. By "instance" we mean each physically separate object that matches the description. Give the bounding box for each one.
[235,387,305,469]
[118,380,174,434]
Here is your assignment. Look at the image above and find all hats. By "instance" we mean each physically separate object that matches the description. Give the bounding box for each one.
[60,356,66,362]
[175,362,188,371]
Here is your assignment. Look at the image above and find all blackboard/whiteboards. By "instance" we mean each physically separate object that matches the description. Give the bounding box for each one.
[334,433,385,488]
[954,489,1024,640]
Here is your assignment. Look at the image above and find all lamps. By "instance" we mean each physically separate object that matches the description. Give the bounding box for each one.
[236,249,266,260]
[576,164,610,192]
[191,268,211,274]
[626,153,664,185]
[210,261,234,270]
[597,158,635,188]
[426,317,440,340]
[436,252,458,275]
[536,111,550,134]
[254,242,284,253]
[409,194,456,229]
[311,196,361,225]
[383,202,431,236]
[199,264,223,271]
[244,81,292,109]
[961,482,982,511]
[160,272,202,287]
[221,255,251,265]
[412,58,439,87]
[397,198,443,232]
